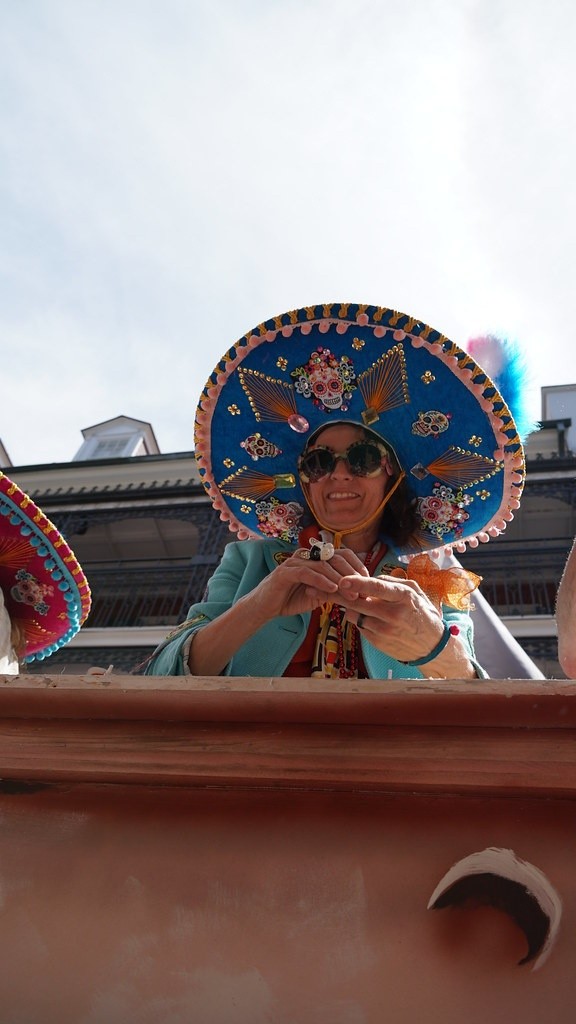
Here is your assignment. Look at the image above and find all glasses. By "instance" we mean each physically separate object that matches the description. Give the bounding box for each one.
[296,439,395,484]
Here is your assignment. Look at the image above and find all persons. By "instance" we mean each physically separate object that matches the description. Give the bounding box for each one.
[141,424,491,684]
[554,537,576,678]
[1,587,25,675]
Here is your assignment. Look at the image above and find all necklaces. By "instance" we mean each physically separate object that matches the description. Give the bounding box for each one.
[334,549,372,679]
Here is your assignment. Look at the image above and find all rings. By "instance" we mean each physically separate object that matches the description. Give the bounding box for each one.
[356,614,367,630]
[297,536,334,560]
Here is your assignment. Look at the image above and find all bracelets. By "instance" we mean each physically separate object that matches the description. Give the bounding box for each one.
[396,617,460,666]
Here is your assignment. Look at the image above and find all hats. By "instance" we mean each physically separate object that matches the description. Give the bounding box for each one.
[193,302,526,562]
[0,471,93,665]
